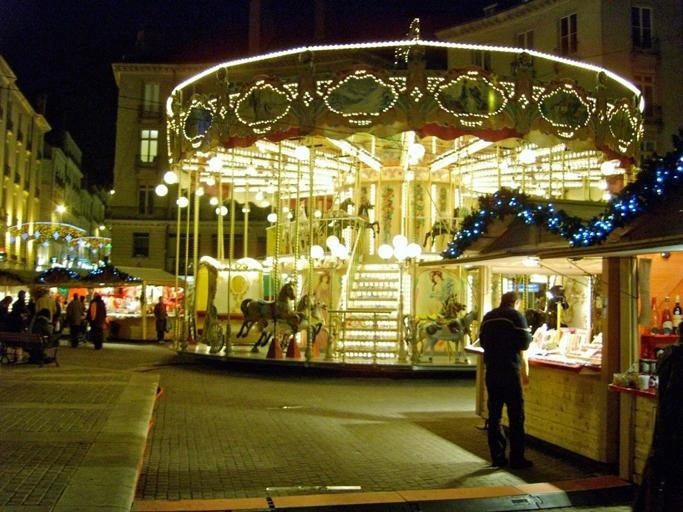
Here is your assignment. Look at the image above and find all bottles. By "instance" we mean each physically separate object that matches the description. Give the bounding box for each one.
[651,294,683,335]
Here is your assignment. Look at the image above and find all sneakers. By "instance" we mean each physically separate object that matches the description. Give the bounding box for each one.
[493,456,533,469]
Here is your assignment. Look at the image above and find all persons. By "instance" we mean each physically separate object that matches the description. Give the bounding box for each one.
[153,296,167,344]
[478,290,535,470]
[630,320,682,512]
[314,273,330,309]
[428,271,458,305]
[0,287,107,364]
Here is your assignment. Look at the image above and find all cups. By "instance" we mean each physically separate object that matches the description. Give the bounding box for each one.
[638,375,650,389]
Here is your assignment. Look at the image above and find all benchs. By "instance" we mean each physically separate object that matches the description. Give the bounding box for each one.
[0,328,61,370]
